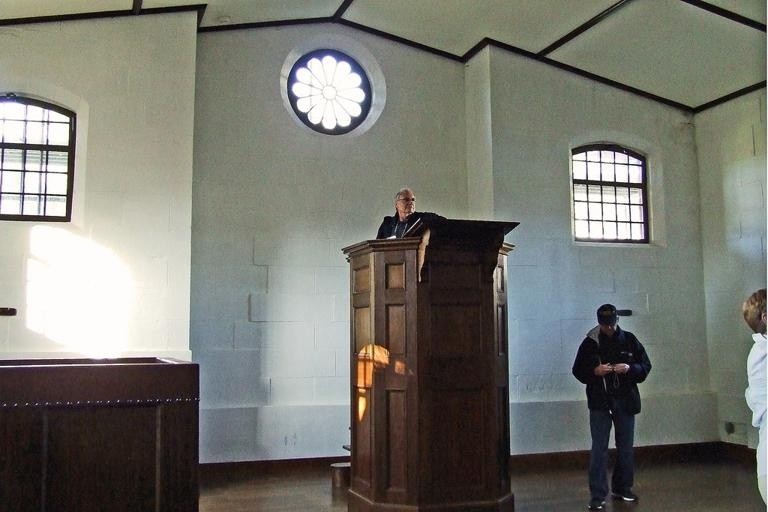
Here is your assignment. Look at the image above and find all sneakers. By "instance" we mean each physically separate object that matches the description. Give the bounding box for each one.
[586,495,606,510]
[611,487,639,504]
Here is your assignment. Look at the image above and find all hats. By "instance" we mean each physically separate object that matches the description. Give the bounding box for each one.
[596,303,618,328]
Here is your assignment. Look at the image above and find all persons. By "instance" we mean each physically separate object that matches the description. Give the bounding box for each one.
[571,303,652,508]
[375,190,431,237]
[741,290,767,511]
